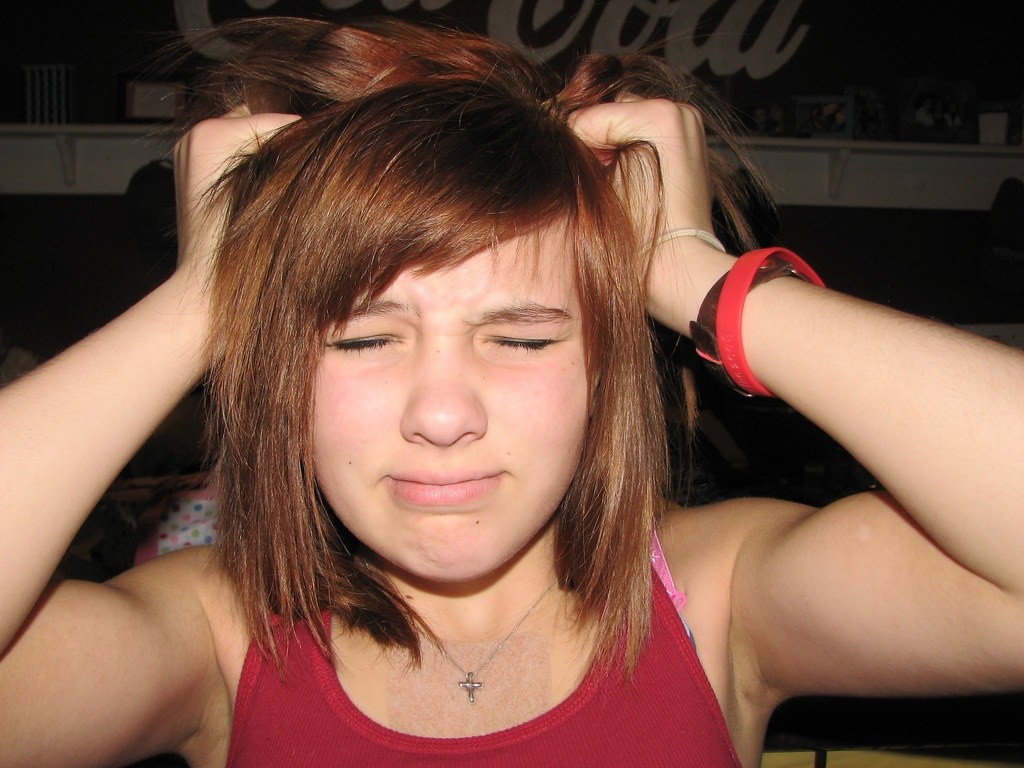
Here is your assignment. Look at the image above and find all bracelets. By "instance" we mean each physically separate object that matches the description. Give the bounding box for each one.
[633,227,824,398]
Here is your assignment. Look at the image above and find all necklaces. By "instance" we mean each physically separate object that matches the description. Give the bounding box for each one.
[360,554,556,702]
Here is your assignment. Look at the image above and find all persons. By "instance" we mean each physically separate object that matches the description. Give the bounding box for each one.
[0,17,1024,768]
